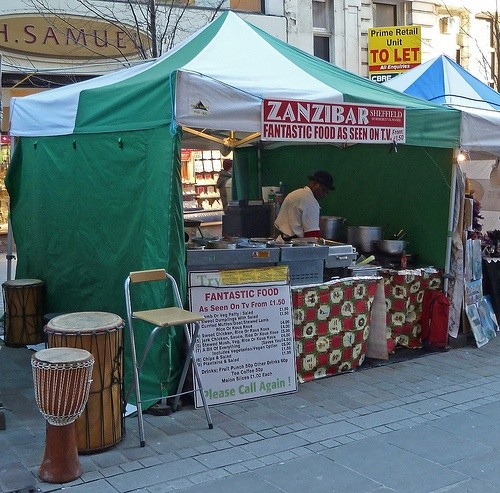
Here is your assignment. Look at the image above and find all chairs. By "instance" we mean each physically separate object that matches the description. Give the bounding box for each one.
[124,269,213,447]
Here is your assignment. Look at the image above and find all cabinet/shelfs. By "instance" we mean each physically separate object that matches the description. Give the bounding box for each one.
[186,149,234,212]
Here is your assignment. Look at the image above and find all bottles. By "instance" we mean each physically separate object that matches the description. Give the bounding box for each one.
[268,189,275,204]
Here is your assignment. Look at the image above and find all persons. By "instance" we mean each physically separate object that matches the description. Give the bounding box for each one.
[216,158,233,212]
[274,171,336,243]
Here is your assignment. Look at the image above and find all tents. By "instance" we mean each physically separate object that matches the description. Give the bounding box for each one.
[1,10,464,418]
[380,53,500,237]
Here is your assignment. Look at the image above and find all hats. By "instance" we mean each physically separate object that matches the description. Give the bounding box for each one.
[307,171,336,190]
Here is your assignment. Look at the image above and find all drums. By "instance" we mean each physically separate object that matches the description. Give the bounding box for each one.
[46,310,128,455]
[30,348,97,485]
[2,279,49,349]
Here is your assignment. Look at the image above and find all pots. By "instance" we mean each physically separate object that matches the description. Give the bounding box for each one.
[347,226,382,253]
[372,240,410,254]
[319,216,346,241]
